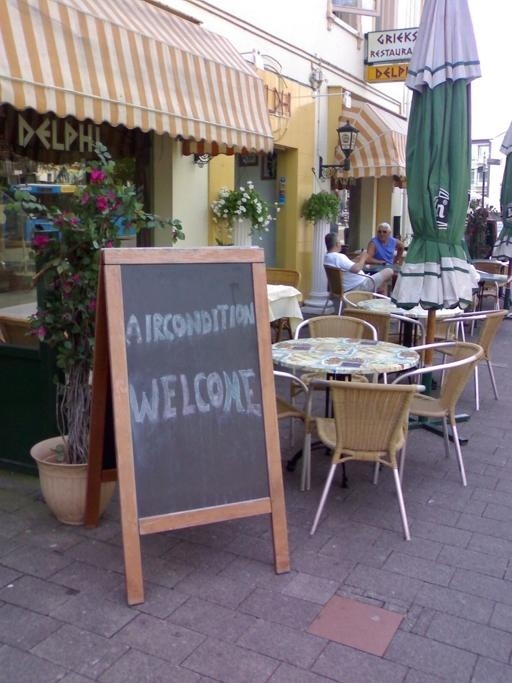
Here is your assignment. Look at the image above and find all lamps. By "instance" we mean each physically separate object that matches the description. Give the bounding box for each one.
[317,117,359,184]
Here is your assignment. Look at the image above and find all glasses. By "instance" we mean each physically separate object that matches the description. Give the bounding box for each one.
[378,230,386,233]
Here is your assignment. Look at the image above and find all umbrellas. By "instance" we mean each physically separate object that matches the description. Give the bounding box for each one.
[388,0,483,408]
[493,120,512,321]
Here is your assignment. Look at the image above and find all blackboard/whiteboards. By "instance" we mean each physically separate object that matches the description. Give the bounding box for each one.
[101,247,283,534]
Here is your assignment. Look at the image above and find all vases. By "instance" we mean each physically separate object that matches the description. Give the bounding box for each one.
[29,432,125,528]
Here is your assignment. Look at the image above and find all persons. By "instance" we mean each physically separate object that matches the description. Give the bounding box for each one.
[322,230,395,300]
[368,221,406,268]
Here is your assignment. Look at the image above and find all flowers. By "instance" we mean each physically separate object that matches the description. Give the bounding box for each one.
[6,141,186,466]
[299,188,349,228]
[210,179,280,242]
[467,198,490,251]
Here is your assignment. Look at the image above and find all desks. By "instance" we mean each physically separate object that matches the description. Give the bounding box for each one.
[271,338,419,473]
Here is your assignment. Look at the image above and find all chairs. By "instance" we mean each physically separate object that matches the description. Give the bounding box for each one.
[321,255,512,414]
[303,380,426,543]
[371,340,485,497]
[273,367,316,492]
[288,315,378,447]
